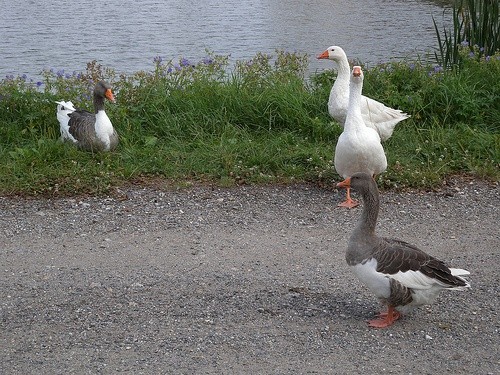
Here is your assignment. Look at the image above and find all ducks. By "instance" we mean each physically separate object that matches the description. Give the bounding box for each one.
[334,65,388,209]
[336,173,472,328]
[316,45,412,187]
[54,81,119,153]
